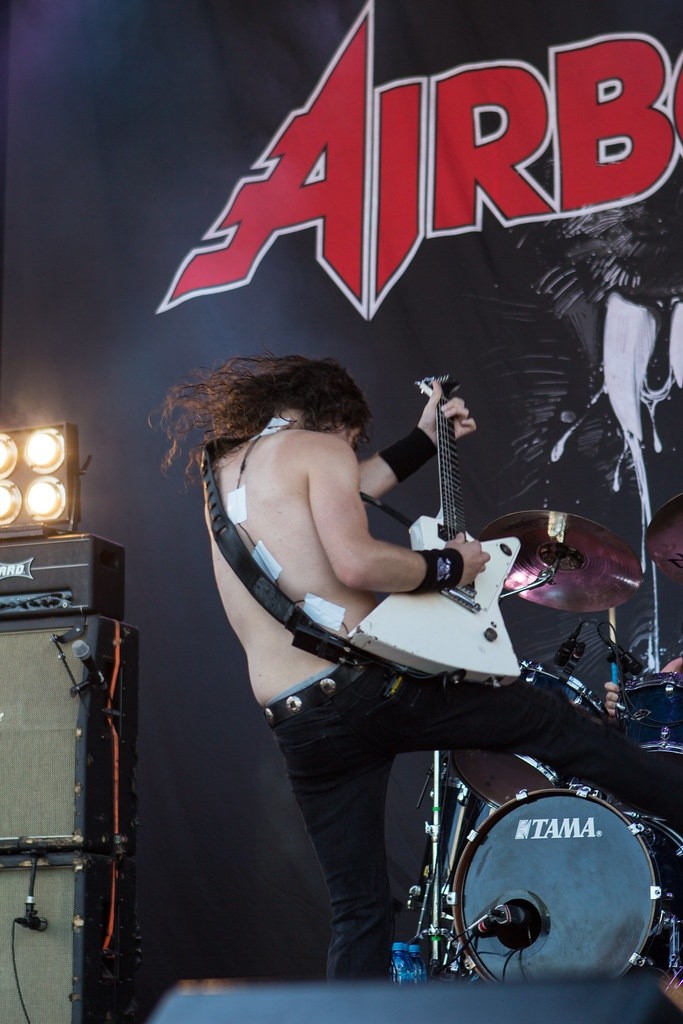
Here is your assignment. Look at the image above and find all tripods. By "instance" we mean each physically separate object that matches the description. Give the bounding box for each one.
[406,749,459,978]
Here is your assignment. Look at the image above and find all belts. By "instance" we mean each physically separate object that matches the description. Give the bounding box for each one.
[263,661,369,732]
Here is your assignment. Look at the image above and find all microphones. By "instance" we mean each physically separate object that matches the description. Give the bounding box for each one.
[598,631,644,676]
[72,640,110,695]
[552,624,583,666]
[14,916,48,932]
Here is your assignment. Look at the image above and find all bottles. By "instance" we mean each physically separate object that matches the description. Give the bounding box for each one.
[389,941,427,988]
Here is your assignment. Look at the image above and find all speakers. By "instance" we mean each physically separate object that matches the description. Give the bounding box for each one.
[0,615,140,854]
[0,852,141,1024]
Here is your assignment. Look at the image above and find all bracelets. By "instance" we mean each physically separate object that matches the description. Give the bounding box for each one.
[401,548,464,595]
[379,426,438,484]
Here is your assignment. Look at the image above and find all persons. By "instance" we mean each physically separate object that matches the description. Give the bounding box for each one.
[601,658,683,716]
[155,356,683,985]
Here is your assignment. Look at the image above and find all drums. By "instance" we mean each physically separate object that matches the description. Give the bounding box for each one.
[449,658,683,991]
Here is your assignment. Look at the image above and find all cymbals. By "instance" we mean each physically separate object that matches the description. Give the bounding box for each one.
[643,492,683,587]
[476,509,643,614]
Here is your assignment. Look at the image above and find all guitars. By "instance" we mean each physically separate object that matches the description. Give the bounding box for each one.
[344,372,524,691]
